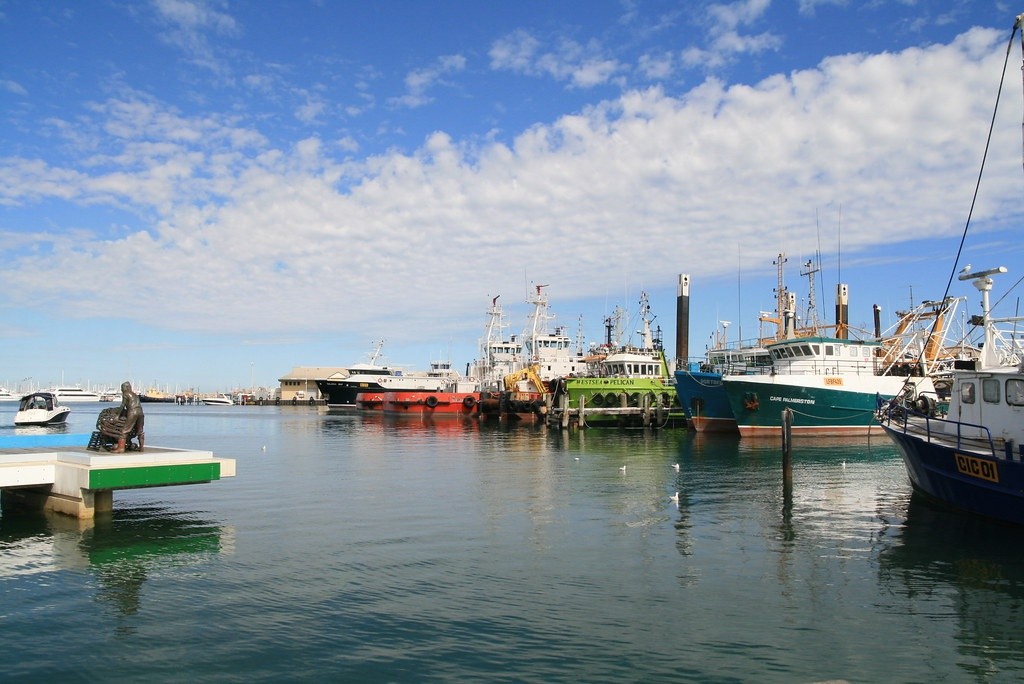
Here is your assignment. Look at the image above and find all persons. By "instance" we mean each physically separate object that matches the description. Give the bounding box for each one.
[110,380,147,453]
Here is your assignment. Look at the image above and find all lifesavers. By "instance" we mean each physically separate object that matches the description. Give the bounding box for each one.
[378,378,382,383]
[592,393,604,406]
[630,392,640,405]
[509,400,532,412]
[469,377,475,382]
[425,396,438,408]
[259,397,263,401]
[605,392,617,405]
[463,395,476,409]
[618,392,630,404]
[917,395,932,417]
[293,397,297,401]
[276,397,280,401]
[310,397,313,401]
[660,392,670,406]
[643,392,655,405]
[549,379,557,392]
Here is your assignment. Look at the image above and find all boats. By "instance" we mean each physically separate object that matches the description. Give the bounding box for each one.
[674,208,971,440]
[315,268,585,410]
[559,287,687,424]
[201,392,235,407]
[14,390,72,426]
[871,262,1023,529]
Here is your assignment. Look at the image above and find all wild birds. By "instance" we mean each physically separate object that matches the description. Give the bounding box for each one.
[572,455,847,502]
[260,444,266,451]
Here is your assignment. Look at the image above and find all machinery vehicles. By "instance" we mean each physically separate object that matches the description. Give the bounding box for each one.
[479,362,552,414]
